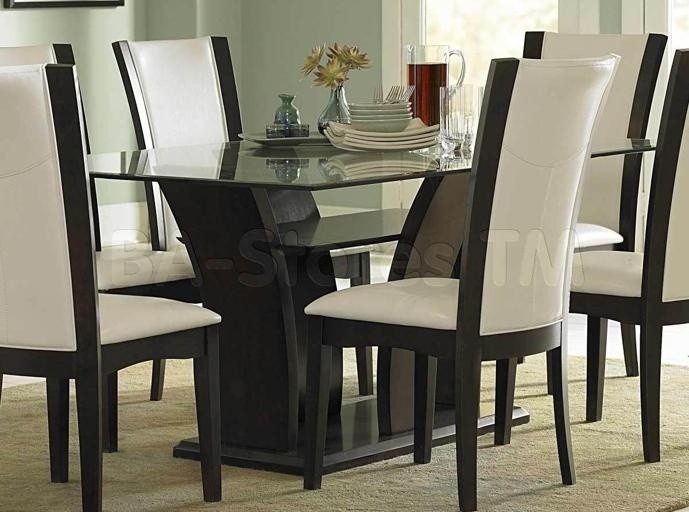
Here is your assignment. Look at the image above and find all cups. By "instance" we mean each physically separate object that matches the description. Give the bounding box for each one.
[440,83,484,162]
[264,121,310,138]
[409,45,464,124]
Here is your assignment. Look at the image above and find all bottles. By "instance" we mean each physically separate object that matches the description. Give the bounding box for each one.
[274,94,300,123]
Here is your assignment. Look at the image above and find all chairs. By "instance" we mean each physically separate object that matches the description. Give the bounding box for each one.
[523,31,670,396]
[2,42,200,453]
[0,64,223,509]
[113,35,376,402]
[493,48,689,465]
[303,48,622,512]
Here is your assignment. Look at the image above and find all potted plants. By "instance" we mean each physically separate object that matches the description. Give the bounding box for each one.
[298,43,372,134]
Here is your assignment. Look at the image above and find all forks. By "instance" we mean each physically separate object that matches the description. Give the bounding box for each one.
[370,80,416,105]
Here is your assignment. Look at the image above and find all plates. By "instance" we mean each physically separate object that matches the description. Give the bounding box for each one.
[324,102,439,150]
[238,123,337,149]
